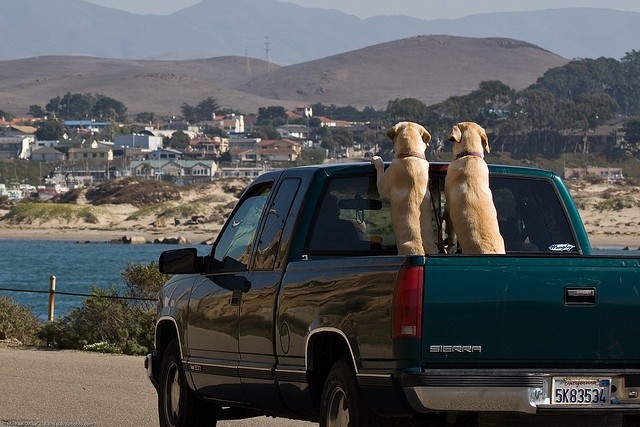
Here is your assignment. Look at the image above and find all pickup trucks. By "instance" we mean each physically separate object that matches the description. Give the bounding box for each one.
[143,160,639,426]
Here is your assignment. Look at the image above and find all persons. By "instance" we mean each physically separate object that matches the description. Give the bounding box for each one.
[317,194,341,223]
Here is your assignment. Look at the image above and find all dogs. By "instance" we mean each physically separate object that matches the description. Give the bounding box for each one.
[443,121,506,255]
[372,121,438,257]
